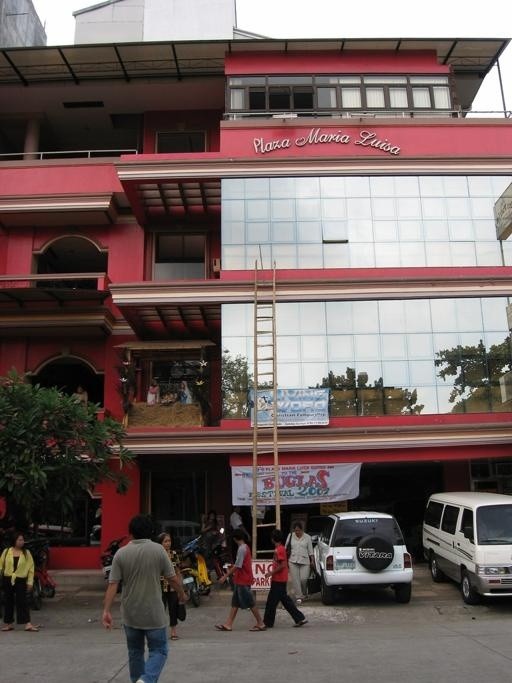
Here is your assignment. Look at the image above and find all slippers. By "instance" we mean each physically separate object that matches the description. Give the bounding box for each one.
[249,625,266,632]
[1,624,14,631]
[293,619,309,627]
[172,635,178,639]
[215,623,232,631]
[24,625,39,632]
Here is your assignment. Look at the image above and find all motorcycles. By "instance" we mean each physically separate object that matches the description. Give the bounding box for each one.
[181,537,211,606]
[30,543,57,611]
[210,529,234,585]
[100,537,126,594]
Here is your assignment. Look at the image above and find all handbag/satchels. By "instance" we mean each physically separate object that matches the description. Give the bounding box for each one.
[307,566,322,594]
[176,604,186,621]
[286,531,292,557]
[0,548,9,589]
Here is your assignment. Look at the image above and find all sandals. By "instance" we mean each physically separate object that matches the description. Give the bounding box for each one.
[296,598,301,603]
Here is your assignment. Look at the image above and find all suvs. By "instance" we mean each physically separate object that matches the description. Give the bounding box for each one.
[313,511,412,606]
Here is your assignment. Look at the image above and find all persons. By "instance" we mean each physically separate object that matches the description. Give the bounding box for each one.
[201,512,218,532]
[229,506,251,541]
[100,515,189,683]
[214,529,266,633]
[155,529,185,641]
[71,387,88,415]
[146,377,160,407]
[284,523,315,605]
[0,534,39,633]
[179,380,192,404]
[262,529,309,628]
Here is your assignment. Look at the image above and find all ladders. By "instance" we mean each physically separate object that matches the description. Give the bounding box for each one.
[251,259,281,604]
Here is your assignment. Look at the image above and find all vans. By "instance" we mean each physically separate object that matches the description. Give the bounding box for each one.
[423,491,512,602]
